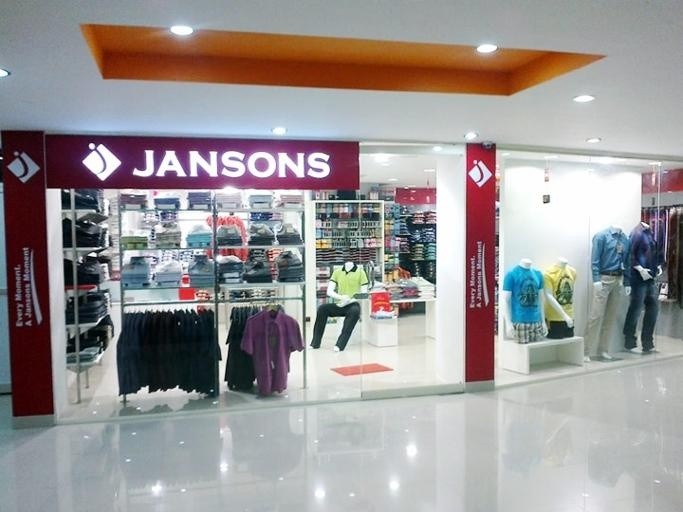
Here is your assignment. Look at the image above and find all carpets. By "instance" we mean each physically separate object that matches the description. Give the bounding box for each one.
[329,363,394,377]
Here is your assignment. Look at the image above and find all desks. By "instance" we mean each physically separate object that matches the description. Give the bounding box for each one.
[360,298,437,346]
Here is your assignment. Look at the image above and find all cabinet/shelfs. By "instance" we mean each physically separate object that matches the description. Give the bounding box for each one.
[117,190,308,292]
[394,212,436,281]
[313,198,386,298]
[64,209,113,374]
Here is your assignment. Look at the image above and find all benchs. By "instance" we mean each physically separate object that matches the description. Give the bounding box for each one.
[501,335,585,374]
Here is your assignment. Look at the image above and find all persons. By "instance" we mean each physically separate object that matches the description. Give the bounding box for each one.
[583,224,632,362]
[503,258,549,344]
[308,261,369,352]
[623,222,664,353]
[543,258,577,339]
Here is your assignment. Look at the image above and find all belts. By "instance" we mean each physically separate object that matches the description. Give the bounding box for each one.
[600,271,622,276]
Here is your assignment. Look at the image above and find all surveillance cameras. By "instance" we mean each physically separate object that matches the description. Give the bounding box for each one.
[484,142,491,148]
